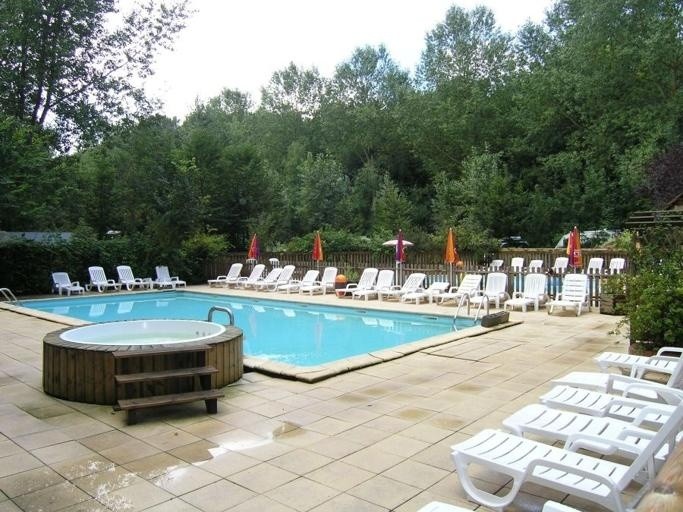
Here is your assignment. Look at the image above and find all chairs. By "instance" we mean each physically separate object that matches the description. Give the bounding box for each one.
[154,265,186,289]
[51,272,85,296]
[85,266,122,293]
[116,265,149,291]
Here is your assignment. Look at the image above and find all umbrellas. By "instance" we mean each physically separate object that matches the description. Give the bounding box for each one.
[312,230,323,270]
[247,233,260,270]
[569,226,583,273]
[445,226,460,285]
[382,240,414,285]
[395,228,405,287]
[567,230,572,254]
